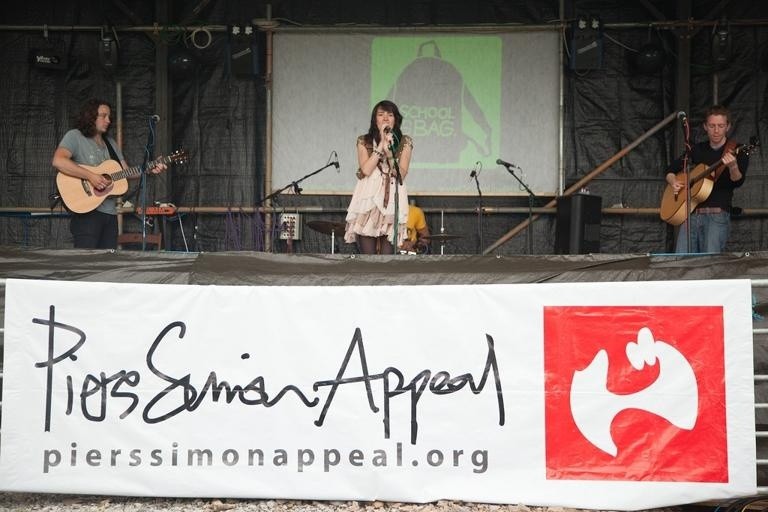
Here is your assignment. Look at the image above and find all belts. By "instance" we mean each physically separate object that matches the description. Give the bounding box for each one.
[690,207,726,215]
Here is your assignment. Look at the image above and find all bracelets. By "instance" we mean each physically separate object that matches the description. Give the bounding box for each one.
[372,148,385,160]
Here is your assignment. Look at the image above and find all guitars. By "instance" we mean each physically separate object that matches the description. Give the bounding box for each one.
[660,136,760,226]
[56,144,194,213]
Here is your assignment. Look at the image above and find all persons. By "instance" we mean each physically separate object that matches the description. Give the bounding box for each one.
[344,101,414,254]
[51,99,168,251]
[395,204,432,254]
[664,105,750,254]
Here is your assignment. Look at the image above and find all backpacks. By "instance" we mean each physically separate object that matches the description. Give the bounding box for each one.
[385,40,492,164]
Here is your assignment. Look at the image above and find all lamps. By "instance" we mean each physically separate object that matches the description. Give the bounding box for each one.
[188,25,224,67]
[94,24,120,72]
[30,25,60,69]
[633,23,661,75]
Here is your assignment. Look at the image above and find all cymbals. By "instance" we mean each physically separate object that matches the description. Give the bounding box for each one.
[421,234,463,240]
[308,221,345,236]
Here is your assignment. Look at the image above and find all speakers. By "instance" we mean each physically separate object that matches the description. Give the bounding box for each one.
[555,194,601,253]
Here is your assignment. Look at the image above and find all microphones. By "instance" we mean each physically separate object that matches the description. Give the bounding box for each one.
[466,164,478,184]
[384,127,390,133]
[678,110,688,124]
[334,153,341,173]
[148,115,160,123]
[496,159,516,168]
